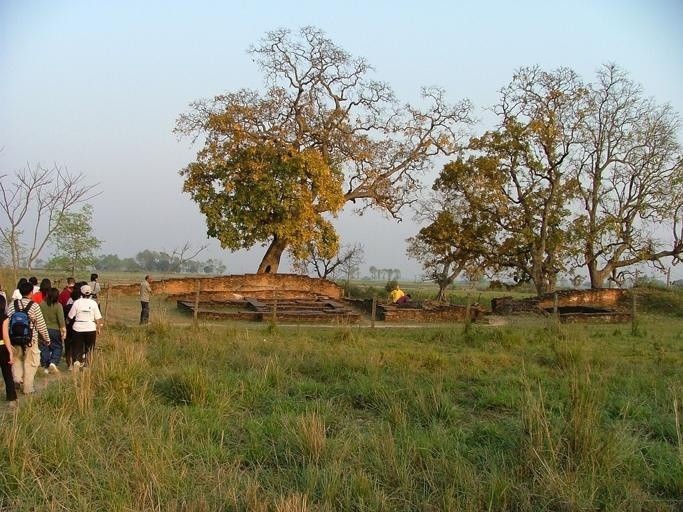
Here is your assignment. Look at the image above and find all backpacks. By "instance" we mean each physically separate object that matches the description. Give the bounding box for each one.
[9,300,34,348]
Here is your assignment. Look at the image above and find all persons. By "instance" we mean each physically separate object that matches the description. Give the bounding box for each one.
[0,272,103,410]
[395,293,412,305]
[388,284,404,303]
[137,274,154,324]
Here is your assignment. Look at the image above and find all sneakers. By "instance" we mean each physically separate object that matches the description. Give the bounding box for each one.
[6,378,36,410]
[44,361,85,375]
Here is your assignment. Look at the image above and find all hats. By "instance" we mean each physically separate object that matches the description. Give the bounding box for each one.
[81,285,93,295]
